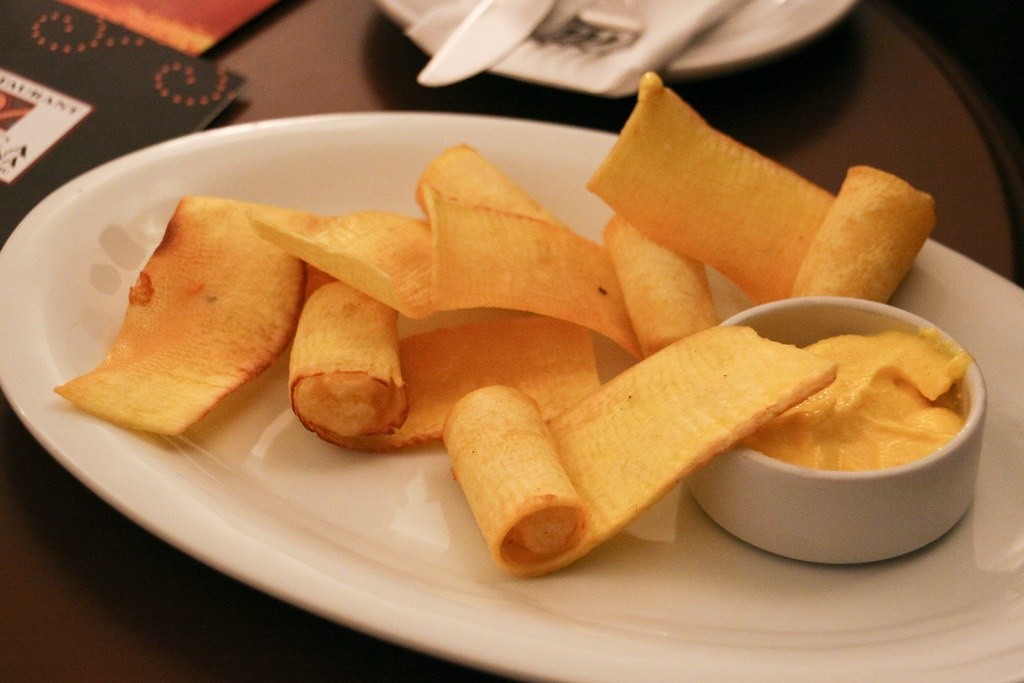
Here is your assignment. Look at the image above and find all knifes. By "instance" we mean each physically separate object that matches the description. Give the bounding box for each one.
[415,0,557,88]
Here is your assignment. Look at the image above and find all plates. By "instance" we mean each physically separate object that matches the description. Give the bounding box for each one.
[0,110,1023,683]
[684,294,987,566]
[374,0,861,95]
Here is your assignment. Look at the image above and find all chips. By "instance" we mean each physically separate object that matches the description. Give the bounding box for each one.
[53,72,935,580]
